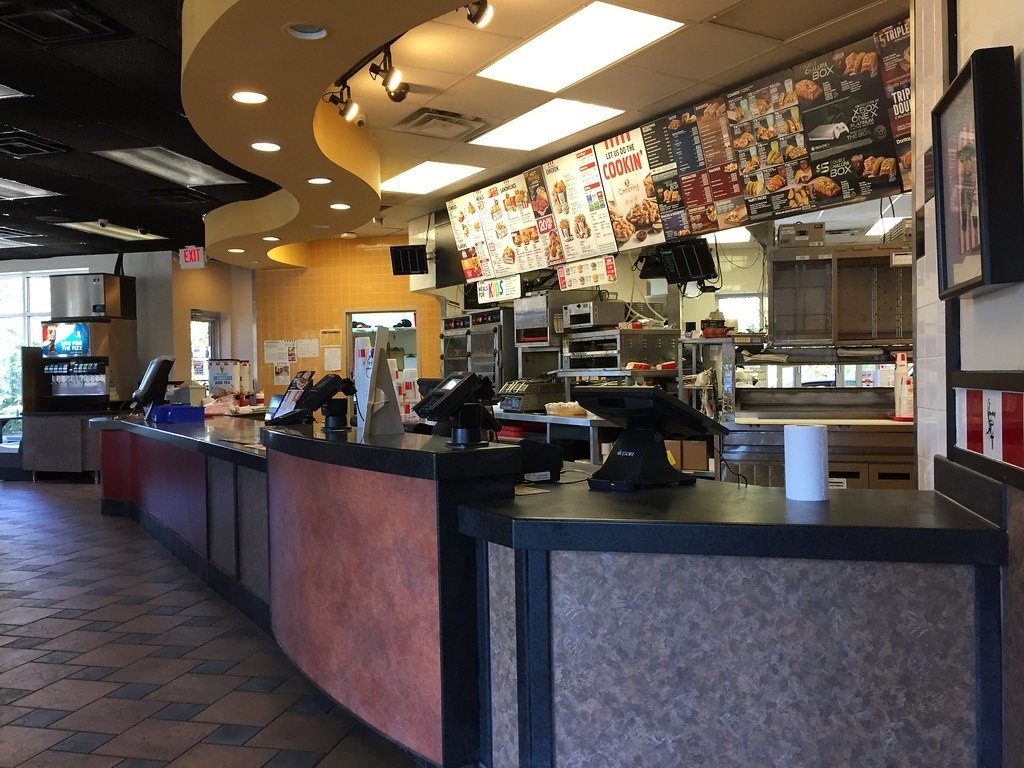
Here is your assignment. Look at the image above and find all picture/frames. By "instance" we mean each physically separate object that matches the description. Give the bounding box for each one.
[930,46,1023,301]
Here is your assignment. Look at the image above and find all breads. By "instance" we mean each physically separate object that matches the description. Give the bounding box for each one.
[663,51,896,224]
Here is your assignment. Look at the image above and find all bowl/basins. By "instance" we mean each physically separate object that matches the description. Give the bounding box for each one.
[627,215,659,228]
[615,224,635,242]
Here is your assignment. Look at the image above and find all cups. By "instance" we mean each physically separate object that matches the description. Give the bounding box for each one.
[771,141,779,152]
[766,115,773,128]
[785,79,792,92]
[750,148,757,157]
[796,135,804,147]
[744,123,752,134]
[791,107,799,120]
[577,221,585,231]
[562,226,570,238]
[256,394,264,406]
[356,337,420,428]
[556,190,567,207]
[740,99,748,112]
[894,353,913,417]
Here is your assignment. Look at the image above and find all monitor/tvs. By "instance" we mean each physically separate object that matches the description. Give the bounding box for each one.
[567,385,729,492]
[40,320,92,360]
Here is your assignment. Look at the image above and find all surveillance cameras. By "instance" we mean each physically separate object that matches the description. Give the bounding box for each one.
[352,112,366,128]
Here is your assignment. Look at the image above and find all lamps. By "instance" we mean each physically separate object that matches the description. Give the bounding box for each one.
[373,217,384,226]
[368,46,402,90]
[464,0,494,28]
[322,81,358,122]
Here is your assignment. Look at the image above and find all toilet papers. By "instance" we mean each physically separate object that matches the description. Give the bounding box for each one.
[783,424,831,502]
